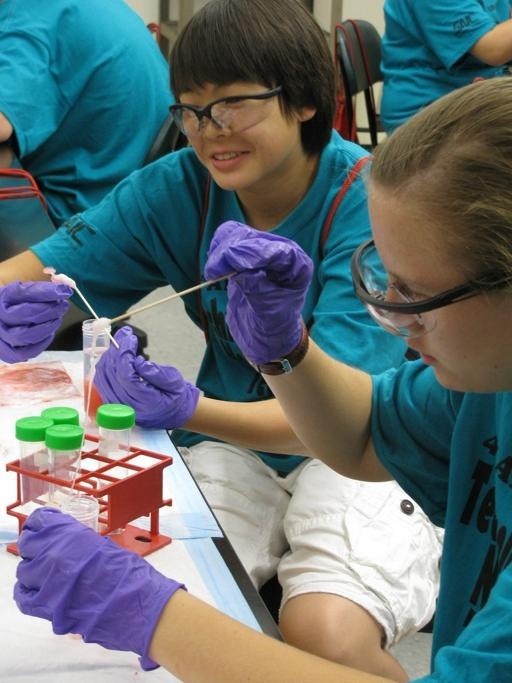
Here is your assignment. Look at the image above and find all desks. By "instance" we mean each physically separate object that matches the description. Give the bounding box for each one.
[1,351,285,678]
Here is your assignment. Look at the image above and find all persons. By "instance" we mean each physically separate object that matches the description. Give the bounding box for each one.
[382,0,512,136]
[12,76,512,681]
[1,0,178,229]
[1,1,444,682]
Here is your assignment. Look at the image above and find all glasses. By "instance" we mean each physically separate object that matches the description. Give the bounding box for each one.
[350,238,512,339]
[169,85,284,137]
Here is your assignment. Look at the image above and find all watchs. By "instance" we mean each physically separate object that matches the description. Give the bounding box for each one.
[256,320,308,376]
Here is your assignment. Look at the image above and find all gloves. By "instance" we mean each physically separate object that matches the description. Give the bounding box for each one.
[0,280,73,364]
[205,221,313,366]
[94,326,200,430]
[13,508,188,671]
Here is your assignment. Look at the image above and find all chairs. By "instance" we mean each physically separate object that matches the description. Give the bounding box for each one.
[144,112,188,165]
